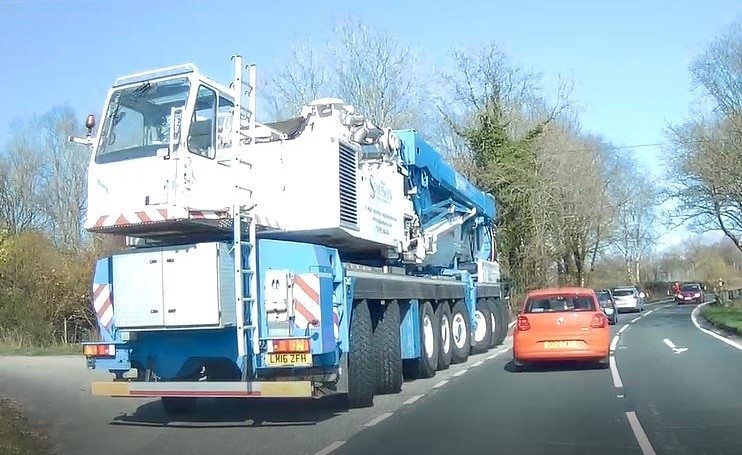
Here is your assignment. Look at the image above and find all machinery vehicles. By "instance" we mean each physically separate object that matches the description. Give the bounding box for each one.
[67,52,510,414]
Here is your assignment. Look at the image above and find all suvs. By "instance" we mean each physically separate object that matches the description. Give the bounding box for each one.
[595,289,619,326]
[610,284,646,313]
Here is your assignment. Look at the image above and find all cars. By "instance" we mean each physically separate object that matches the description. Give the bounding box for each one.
[675,283,705,305]
[513,286,611,372]
